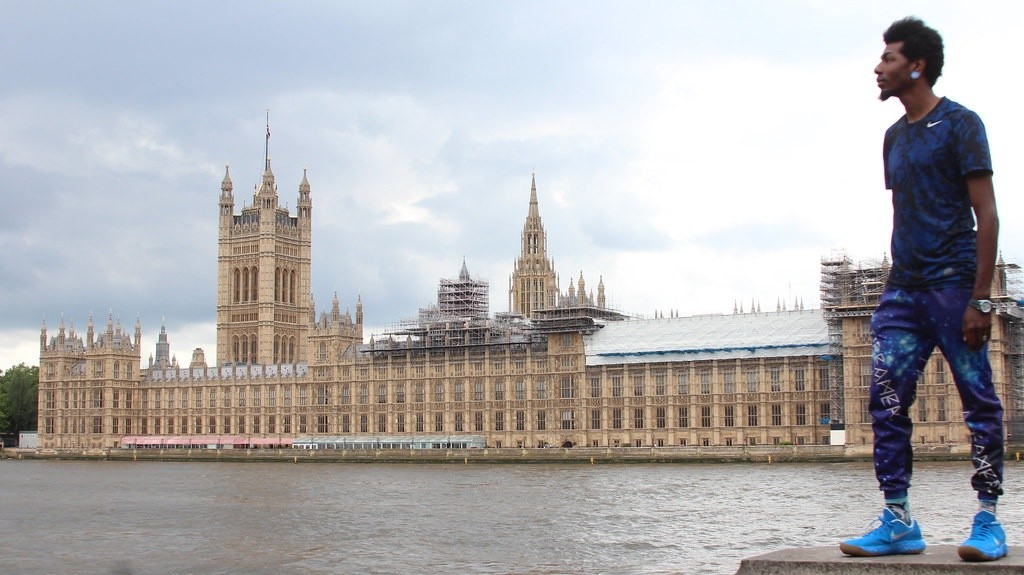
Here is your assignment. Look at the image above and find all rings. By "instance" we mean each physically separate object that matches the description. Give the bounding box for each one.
[983,336,987,342]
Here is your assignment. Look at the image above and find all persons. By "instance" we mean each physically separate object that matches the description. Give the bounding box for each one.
[838,18,1007,560]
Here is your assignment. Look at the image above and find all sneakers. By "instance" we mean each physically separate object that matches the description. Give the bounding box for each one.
[840,507,925,557]
[958,510,1008,561]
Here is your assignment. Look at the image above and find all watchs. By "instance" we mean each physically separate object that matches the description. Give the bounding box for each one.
[969,299,993,313]
